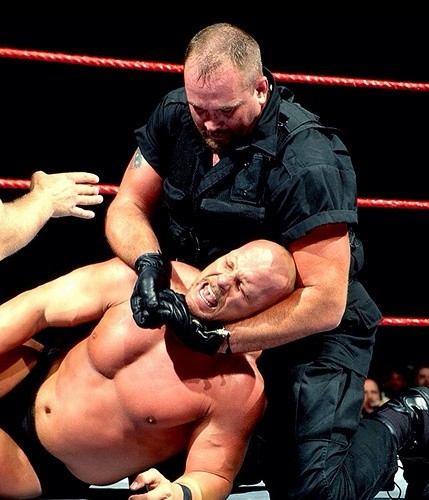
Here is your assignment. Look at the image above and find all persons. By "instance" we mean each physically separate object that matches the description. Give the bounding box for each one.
[0,170,103,260]
[103,22,429,500]
[364,366,429,414]
[0,241,297,500]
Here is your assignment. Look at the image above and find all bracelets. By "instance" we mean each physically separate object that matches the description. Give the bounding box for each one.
[177,483,192,500]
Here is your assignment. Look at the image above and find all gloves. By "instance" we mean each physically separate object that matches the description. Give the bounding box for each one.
[130,253,231,357]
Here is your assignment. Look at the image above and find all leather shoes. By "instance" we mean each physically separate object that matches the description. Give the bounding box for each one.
[371,384,429,500]
